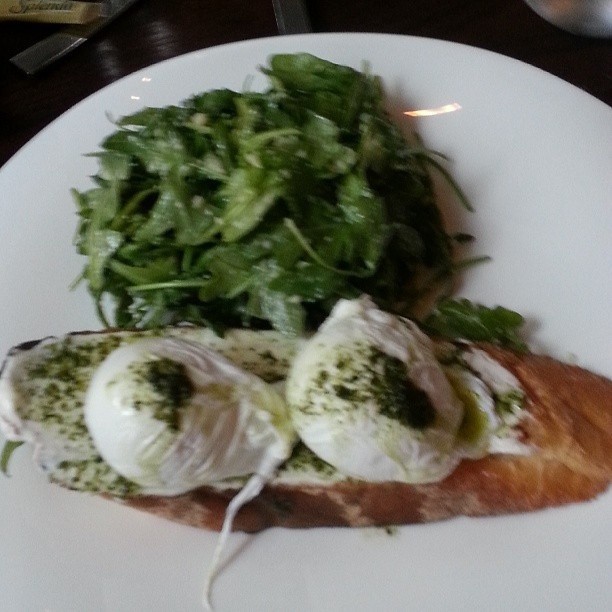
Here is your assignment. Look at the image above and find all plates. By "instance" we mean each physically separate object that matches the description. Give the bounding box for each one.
[0,33,612,612]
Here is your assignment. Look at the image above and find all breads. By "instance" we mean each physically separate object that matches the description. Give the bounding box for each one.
[1,328,611,533]
